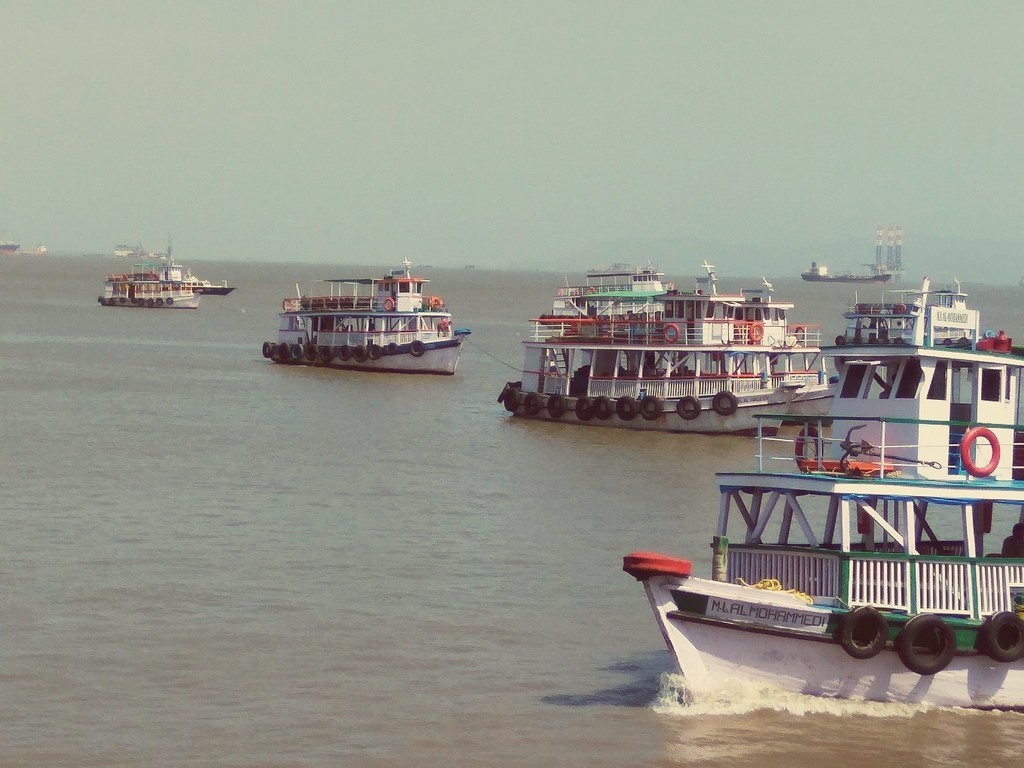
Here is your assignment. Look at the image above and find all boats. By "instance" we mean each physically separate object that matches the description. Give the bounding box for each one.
[498,259,837,440]
[0,238,24,255]
[623,272,1023,718]
[262,256,473,376]
[800,259,893,285]
[29,244,50,256]
[99,230,238,310]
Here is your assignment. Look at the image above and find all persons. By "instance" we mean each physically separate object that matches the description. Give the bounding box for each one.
[369,319,376,332]
[618,311,639,332]
[879,373,902,399]
[1001,522,1024,557]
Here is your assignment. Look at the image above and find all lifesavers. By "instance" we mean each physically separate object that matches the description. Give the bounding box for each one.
[838,606,889,659]
[429,297,442,309]
[713,391,738,416]
[794,326,804,342]
[961,427,1000,478]
[897,614,956,676]
[97,296,173,306]
[749,322,764,342]
[662,322,680,343]
[383,297,395,312]
[897,303,907,315]
[795,426,825,471]
[676,396,700,421]
[262,340,426,363]
[497,381,663,420]
[539,311,664,325]
[283,299,293,311]
[983,611,1024,663]
[300,297,370,308]
